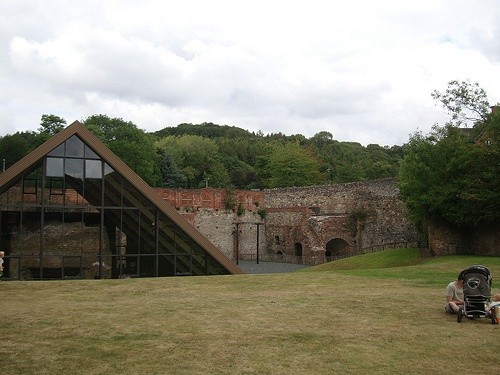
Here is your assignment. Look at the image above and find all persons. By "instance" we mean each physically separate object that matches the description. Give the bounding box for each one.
[444,270,464,314]
[489,293,500,323]
[0,251,4,279]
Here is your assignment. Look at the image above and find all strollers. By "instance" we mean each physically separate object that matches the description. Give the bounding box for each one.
[456,263,496,325]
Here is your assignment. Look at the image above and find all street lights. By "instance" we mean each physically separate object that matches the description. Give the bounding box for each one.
[252,222,264,264]
[231,221,242,265]
